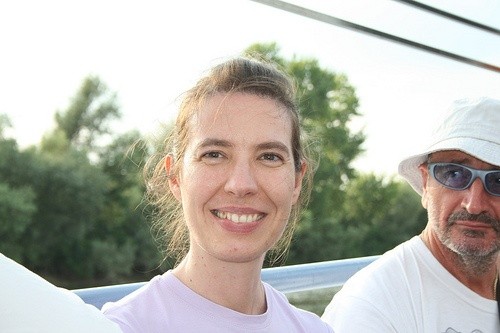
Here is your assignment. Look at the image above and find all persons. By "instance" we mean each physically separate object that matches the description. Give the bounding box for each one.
[320,98,500,333]
[100,58,332,333]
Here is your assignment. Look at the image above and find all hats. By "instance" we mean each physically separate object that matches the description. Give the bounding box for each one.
[398,98,500,195]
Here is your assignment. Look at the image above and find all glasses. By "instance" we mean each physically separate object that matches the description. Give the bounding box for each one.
[426,160,500,195]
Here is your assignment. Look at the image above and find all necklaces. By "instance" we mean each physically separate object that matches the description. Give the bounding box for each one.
[494,268,498,301]
